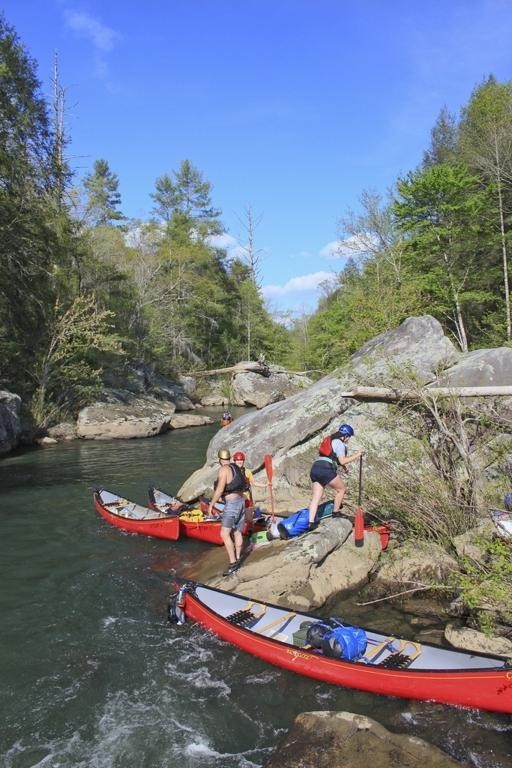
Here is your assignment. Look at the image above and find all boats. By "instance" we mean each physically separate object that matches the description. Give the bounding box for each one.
[94,488,180,540]
[173,578,512,714]
[201,498,282,536]
[148,486,227,546]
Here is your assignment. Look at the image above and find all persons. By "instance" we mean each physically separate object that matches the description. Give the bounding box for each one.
[233,452,272,536]
[208,448,246,576]
[223,408,231,420]
[308,423,364,531]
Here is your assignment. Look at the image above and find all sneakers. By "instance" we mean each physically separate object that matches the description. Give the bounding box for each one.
[223,562,241,576]
[309,522,314,530]
[332,511,347,518]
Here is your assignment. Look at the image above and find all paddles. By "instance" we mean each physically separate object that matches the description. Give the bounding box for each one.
[355,455,363,541]
[265,455,275,522]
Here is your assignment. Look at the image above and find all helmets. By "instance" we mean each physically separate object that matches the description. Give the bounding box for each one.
[218,450,230,460]
[234,452,245,461]
[339,425,353,437]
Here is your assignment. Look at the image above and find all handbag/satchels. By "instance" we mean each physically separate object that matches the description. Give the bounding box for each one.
[307,616,367,660]
[278,509,316,539]
